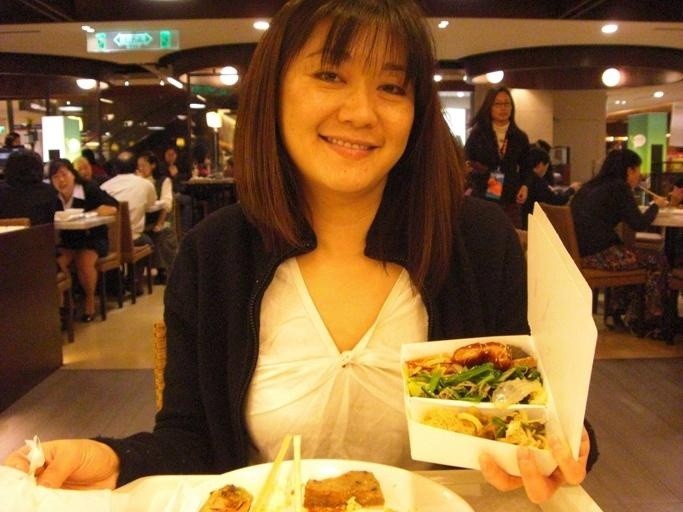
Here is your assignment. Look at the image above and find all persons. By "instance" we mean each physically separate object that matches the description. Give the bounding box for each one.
[534,139,555,186]
[3,132,27,150]
[463,85,531,231]
[193,155,211,179]
[223,157,233,178]
[570,149,671,340]
[72,156,92,182]
[4,0,600,512]
[138,155,178,288]
[100,151,156,295]
[161,148,193,231]
[522,149,581,214]
[48,159,119,322]
[665,179,683,269]
[82,148,108,182]
[0,150,64,227]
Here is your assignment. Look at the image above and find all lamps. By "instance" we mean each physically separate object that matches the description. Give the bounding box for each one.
[205,110,222,131]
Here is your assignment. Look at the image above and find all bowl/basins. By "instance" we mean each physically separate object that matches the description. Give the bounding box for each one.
[66,207,84,216]
[55,211,70,220]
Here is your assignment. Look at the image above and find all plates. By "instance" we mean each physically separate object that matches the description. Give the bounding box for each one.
[173,457,484,512]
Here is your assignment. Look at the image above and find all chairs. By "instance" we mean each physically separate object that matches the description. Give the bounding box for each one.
[57,271,74,342]
[121,201,153,305]
[539,202,646,340]
[96,202,123,321]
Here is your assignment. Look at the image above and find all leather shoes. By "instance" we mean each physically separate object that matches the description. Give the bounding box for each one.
[82,308,95,323]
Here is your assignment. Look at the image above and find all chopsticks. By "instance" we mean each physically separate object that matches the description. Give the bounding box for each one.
[639,185,670,205]
[249,432,306,512]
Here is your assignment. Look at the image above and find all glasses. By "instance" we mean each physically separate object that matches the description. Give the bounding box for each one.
[491,100,513,109]
